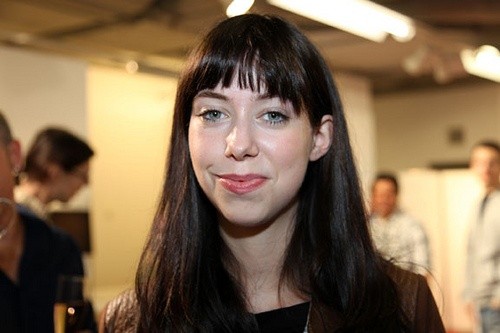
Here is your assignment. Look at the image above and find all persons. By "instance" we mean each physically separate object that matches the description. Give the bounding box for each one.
[97,14,446,333]
[366,175,429,280]
[458,142,500,333]
[0,113,94,333]
